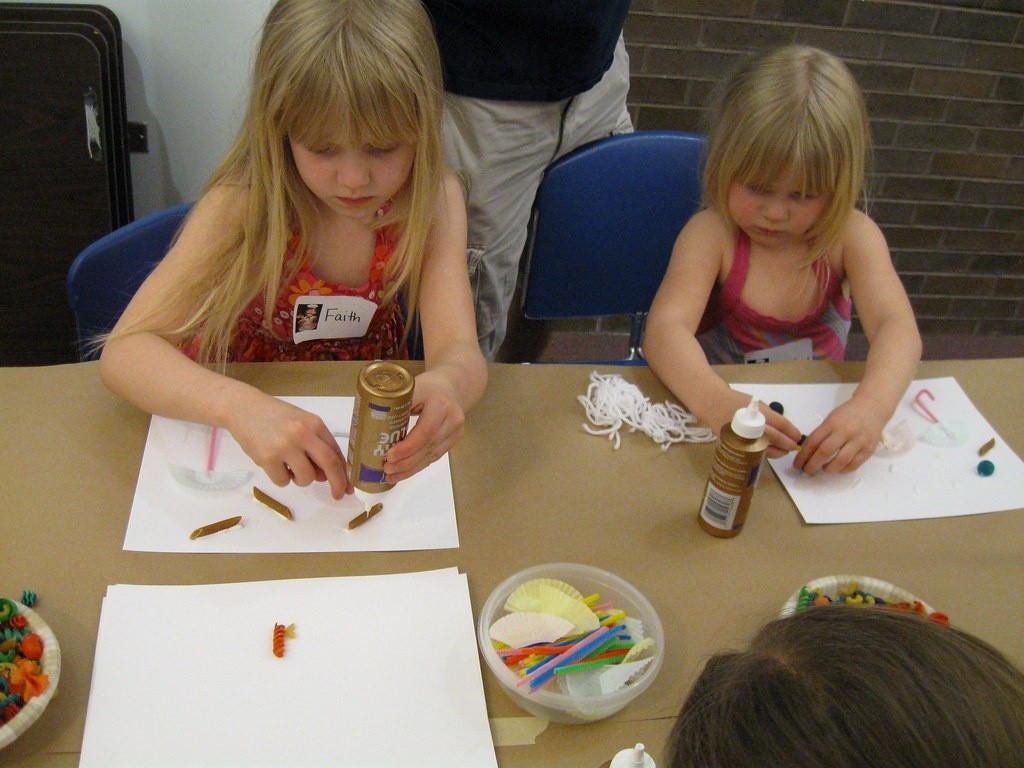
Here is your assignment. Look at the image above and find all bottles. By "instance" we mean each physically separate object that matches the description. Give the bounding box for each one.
[697,395,769,538]
[599,743,656,768]
[344,360,416,512]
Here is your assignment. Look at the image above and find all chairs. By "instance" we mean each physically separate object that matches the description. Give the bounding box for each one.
[522,133,713,363]
[70,200,198,363]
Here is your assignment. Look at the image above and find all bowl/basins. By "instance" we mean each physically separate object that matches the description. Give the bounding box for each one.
[780,573,949,629]
[477,562,665,725]
[0,598,62,750]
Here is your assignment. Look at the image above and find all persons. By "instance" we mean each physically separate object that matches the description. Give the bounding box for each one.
[644,47,922,476]
[665,606,1024,768]
[418,1,635,361]
[99,0,489,500]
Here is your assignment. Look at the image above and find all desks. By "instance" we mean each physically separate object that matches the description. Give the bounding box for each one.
[0,354,1024,767]
